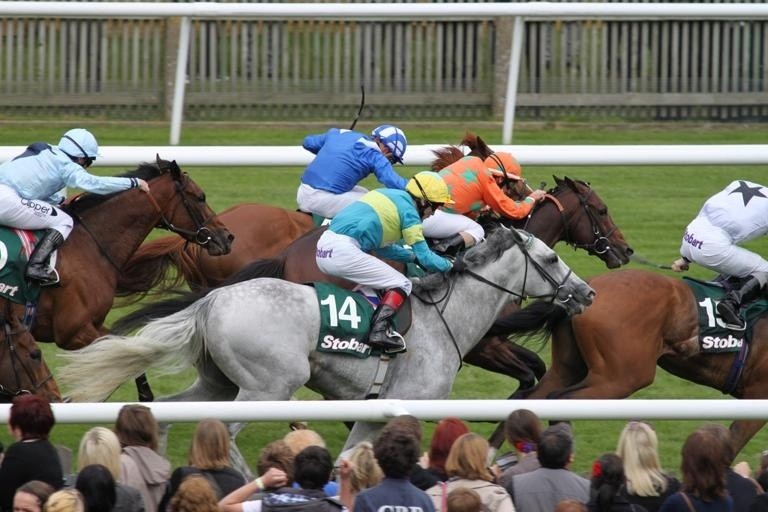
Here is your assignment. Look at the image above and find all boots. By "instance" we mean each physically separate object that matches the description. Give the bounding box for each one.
[26,229,63,288]
[717,275,760,327]
[367,287,406,354]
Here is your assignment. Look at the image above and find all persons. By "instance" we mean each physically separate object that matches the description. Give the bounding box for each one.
[0,127,150,285]
[421,151,546,258]
[672,178,767,326]
[315,168,454,352]
[296,125,407,219]
[0,393,768,512]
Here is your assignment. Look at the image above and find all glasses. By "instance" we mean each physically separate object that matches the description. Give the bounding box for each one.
[65,133,92,167]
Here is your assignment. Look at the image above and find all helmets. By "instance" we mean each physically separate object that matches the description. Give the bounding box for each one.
[485,153,522,181]
[58,127,100,160]
[372,125,406,167]
[406,172,454,205]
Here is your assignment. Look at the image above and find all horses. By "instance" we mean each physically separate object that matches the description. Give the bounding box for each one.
[0,153,235,402]
[109,175,633,472]
[484,267,768,473]
[112,131,534,311]
[54,222,599,487]
[2,295,64,402]
